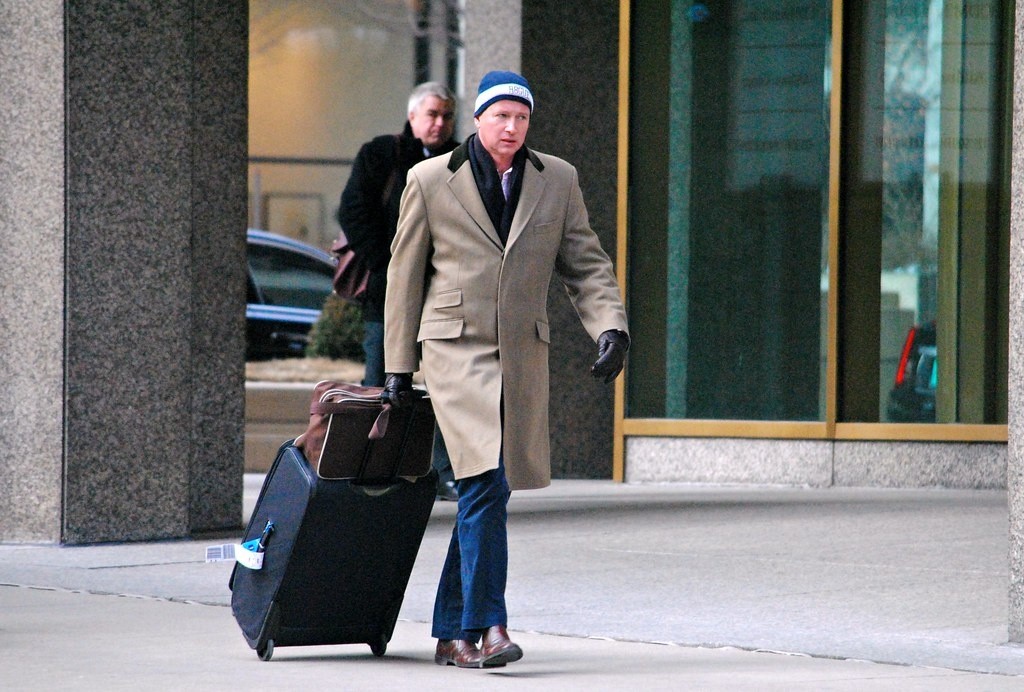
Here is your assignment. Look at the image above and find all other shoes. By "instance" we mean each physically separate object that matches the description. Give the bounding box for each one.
[436,480,459,502]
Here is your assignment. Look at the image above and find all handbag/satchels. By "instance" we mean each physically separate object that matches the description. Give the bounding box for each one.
[293,380,437,480]
[331,233,373,303]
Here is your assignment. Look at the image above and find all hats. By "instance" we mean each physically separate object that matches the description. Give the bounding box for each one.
[473,71,534,116]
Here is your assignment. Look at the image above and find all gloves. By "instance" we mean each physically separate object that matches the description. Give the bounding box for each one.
[591,330,630,384]
[381,374,414,417]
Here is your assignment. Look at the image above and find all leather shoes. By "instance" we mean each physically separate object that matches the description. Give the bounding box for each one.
[479,625,523,669]
[434,640,481,668]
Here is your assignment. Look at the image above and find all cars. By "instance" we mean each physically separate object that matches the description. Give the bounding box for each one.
[243,228,340,363]
[889,320,938,425]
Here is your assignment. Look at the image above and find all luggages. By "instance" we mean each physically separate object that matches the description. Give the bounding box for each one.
[229,392,439,661]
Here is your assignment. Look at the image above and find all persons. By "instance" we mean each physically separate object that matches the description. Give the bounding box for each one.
[384,72,631,668]
[332,81,464,501]
